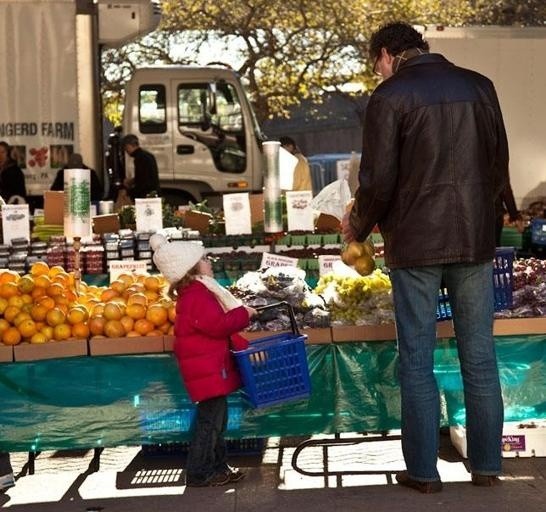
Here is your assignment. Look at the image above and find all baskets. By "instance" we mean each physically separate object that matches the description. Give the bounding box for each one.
[433,242,519,323]
[227,299,315,408]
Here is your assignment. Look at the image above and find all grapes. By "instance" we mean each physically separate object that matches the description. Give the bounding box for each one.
[226,268,398,331]
[493,257,546,318]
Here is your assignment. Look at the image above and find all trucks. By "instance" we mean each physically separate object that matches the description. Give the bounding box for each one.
[0,1,263,209]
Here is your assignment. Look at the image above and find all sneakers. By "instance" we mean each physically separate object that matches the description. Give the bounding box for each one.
[396,469,442,494]
[469,468,497,485]
[184,466,246,487]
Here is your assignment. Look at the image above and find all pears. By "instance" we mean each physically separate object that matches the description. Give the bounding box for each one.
[341,241,375,275]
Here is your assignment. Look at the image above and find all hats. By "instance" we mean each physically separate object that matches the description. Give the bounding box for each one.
[147,234,207,288]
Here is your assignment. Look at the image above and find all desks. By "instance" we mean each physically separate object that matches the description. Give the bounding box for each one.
[0,334,546,512]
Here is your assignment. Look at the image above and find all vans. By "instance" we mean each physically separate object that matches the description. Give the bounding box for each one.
[305,149,365,201]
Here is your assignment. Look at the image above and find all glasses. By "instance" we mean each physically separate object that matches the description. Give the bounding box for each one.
[371,52,384,78]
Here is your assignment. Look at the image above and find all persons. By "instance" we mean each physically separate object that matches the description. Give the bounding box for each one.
[0,141,27,205]
[342,19,511,493]
[495,183,524,247]
[118,134,162,202]
[278,136,313,191]
[50,153,103,200]
[148,233,259,487]
